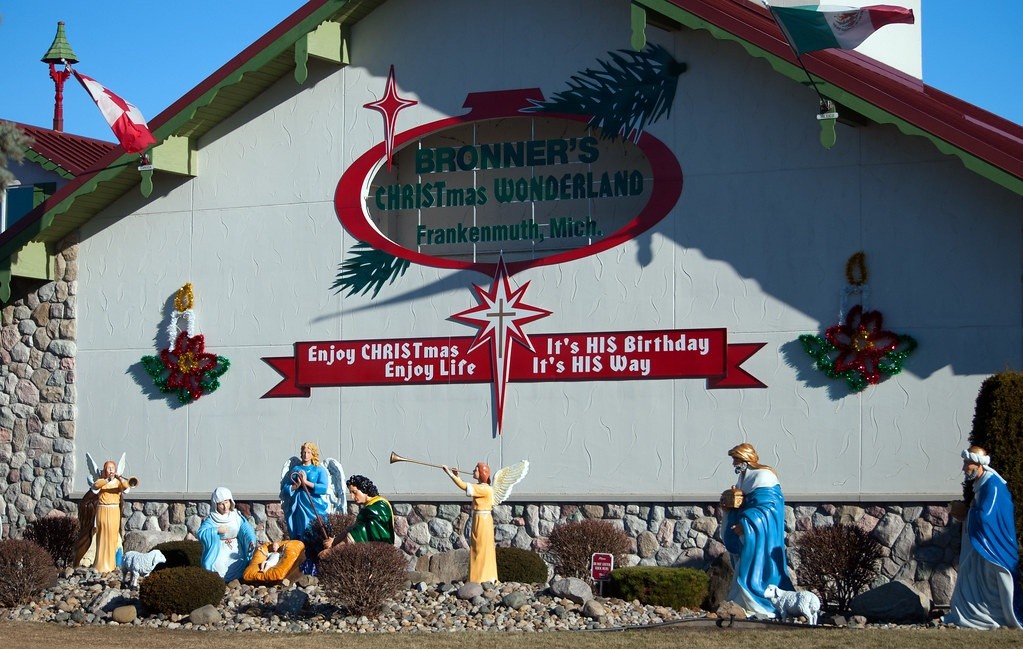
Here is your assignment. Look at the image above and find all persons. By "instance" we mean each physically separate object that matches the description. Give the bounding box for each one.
[941,446,1023,631]
[280,442,328,576]
[318,474,395,559]
[257,541,281,573]
[443,463,498,583]
[722,443,797,618]
[196,487,258,582]
[92,461,129,572]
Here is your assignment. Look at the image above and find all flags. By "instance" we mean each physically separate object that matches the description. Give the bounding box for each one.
[72,68,158,154]
[766,3,915,59]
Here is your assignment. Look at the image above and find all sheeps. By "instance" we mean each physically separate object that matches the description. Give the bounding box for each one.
[763,583,821,628]
